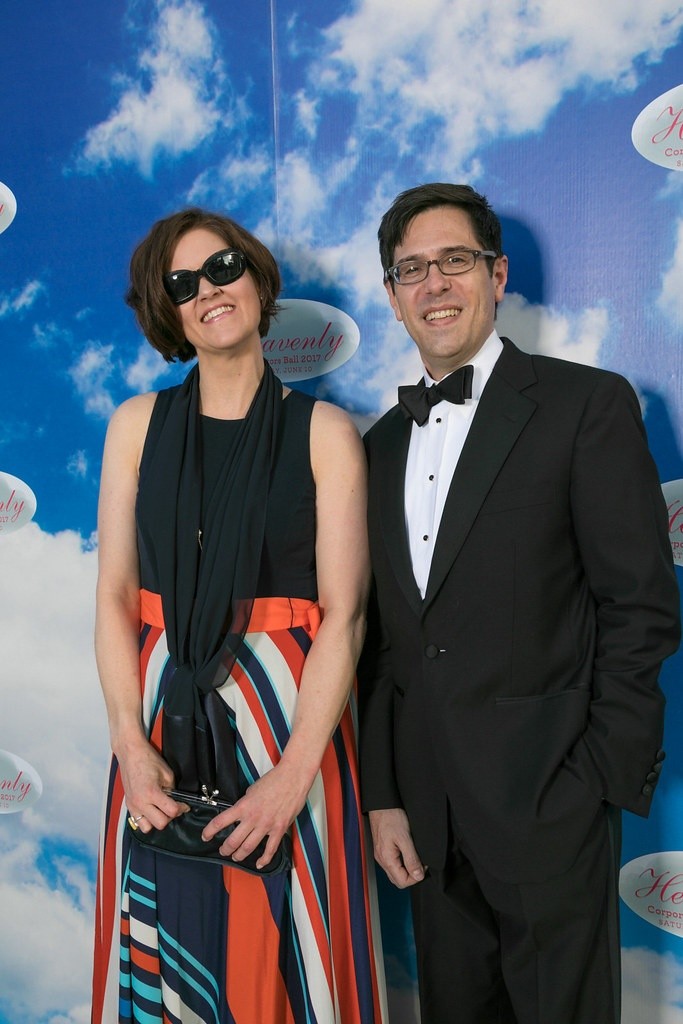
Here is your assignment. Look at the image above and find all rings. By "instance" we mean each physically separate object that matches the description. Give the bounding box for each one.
[128,816,138,831]
[133,815,144,823]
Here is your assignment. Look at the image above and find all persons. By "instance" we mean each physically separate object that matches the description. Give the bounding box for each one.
[356,182,683,1024]
[90,209,388,1024]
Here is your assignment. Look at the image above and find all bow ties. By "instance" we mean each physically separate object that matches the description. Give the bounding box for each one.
[399,364,473,428]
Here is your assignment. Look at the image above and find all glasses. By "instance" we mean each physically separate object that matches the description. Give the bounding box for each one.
[384,250,499,284]
[164,248,247,306]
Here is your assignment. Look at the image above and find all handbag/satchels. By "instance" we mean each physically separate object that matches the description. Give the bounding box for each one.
[130,785,291,877]
